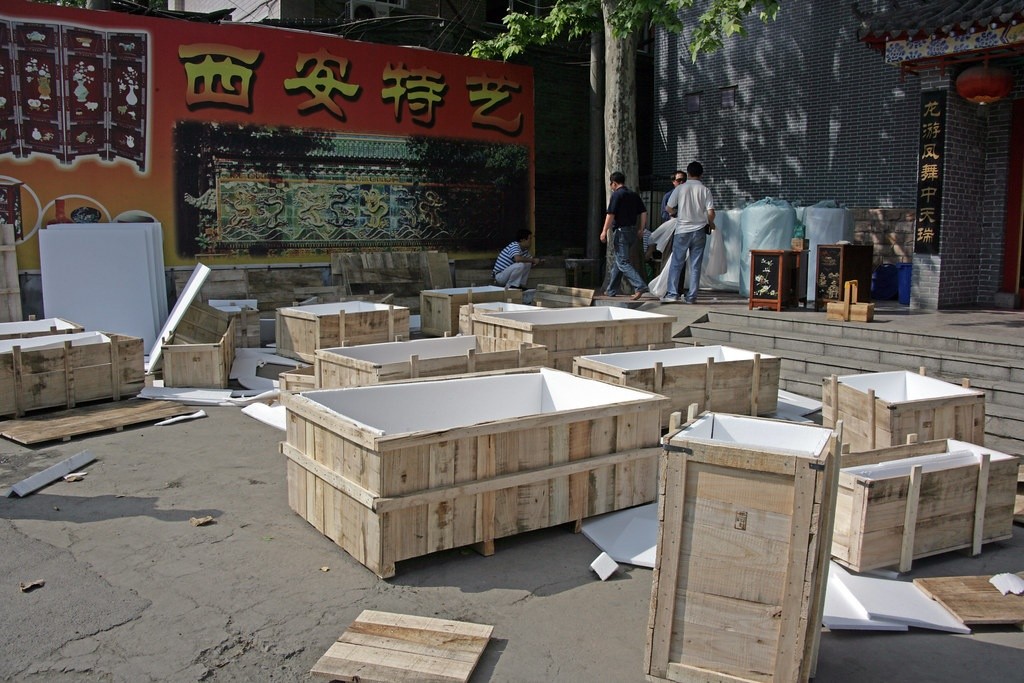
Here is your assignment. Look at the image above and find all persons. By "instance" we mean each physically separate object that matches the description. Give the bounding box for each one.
[661,161,715,304]
[492,229,544,291]
[600,171,648,300]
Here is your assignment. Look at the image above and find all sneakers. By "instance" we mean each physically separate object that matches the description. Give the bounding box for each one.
[660,292,678,302]
[686,295,695,303]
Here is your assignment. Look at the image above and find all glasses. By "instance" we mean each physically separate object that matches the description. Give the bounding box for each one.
[675,179,686,182]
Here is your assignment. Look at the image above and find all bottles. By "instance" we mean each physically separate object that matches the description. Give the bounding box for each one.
[47,195,74,224]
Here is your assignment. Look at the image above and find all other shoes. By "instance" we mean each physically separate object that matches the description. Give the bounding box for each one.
[519,286,528,290]
[604,291,609,297]
[510,286,526,291]
[632,291,642,299]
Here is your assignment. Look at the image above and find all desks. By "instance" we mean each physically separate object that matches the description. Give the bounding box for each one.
[815,244,873,311]
[749,250,804,311]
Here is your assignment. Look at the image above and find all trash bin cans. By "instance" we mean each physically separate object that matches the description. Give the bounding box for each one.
[895,262,912,305]
[564,258,600,290]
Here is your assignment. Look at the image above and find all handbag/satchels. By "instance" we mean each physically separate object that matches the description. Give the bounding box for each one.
[705,224,711,235]
